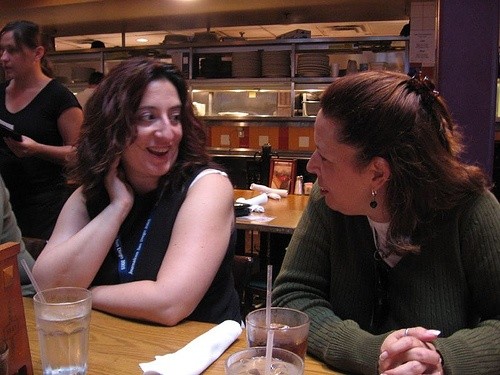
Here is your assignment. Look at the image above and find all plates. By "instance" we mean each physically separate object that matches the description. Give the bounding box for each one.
[262,51,291,78]
[218,112,249,116]
[232,52,261,78]
[56,76,68,84]
[71,67,96,81]
[297,54,330,77]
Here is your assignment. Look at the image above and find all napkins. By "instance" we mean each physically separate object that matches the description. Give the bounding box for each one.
[139,317,244,375]
[235,181,294,214]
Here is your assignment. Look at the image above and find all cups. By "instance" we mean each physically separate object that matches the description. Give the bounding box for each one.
[33,287,92,375]
[245,307,310,363]
[331,63,339,77]
[225,347,304,375]
[304,183,313,194]
[346,59,358,75]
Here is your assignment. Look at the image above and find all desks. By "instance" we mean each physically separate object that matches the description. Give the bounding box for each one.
[23,295,342,375]
[234,189,310,254]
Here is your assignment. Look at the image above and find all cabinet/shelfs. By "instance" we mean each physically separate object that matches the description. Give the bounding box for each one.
[48,34,408,123]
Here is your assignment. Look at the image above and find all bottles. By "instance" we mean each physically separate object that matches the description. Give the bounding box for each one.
[294,175,303,195]
[418,71,423,82]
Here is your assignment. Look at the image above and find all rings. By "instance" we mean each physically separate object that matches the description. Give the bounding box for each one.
[404,328,409,336]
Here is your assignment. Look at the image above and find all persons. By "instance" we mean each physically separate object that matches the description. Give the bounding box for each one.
[268,70,500,375]
[0,18,107,299]
[33,56,244,329]
